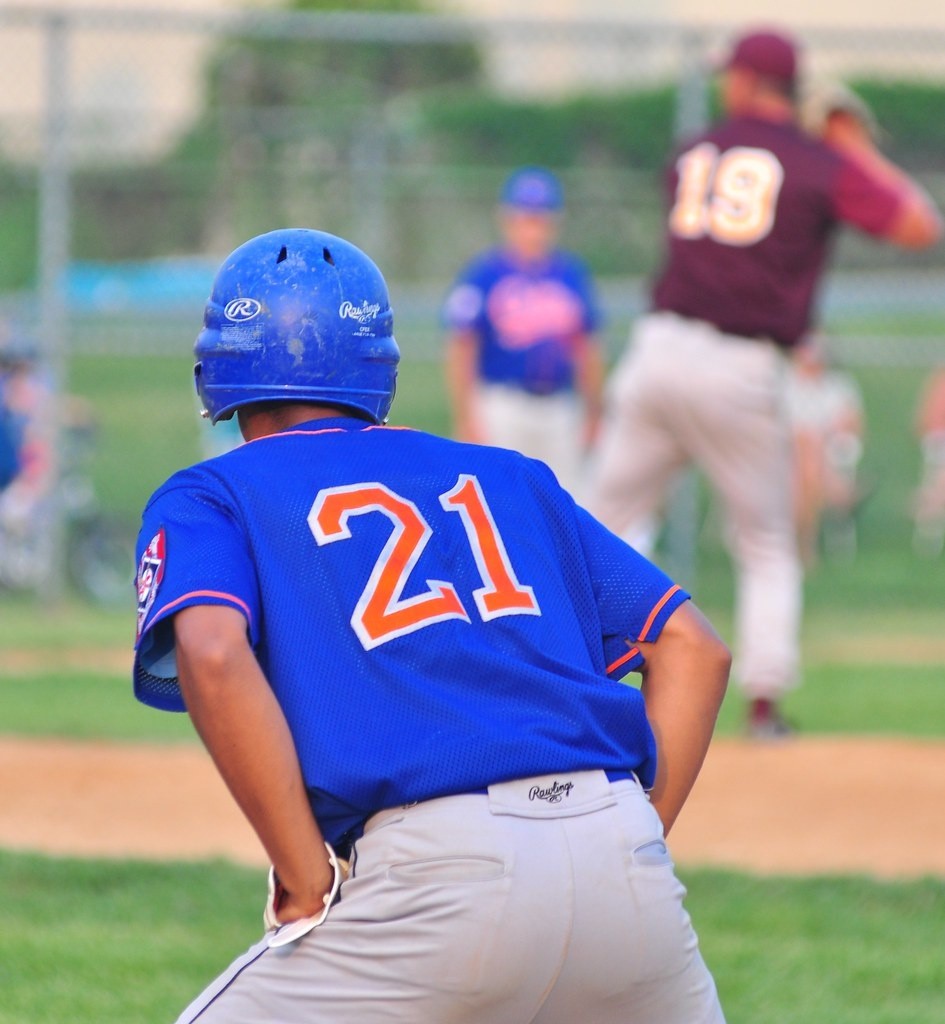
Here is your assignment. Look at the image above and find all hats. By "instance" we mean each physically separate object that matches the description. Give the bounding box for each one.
[500,166,564,209]
[710,34,796,88]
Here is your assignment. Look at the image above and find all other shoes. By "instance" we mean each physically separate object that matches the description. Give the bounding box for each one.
[749,714,799,740]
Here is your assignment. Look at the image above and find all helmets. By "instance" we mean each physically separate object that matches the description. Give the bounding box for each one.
[193,228,400,424]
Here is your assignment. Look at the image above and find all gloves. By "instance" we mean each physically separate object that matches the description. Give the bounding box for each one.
[263,841,350,947]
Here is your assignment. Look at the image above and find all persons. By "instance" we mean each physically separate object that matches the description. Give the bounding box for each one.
[130,224,737,1023]
[577,25,944,747]
[436,165,609,501]
